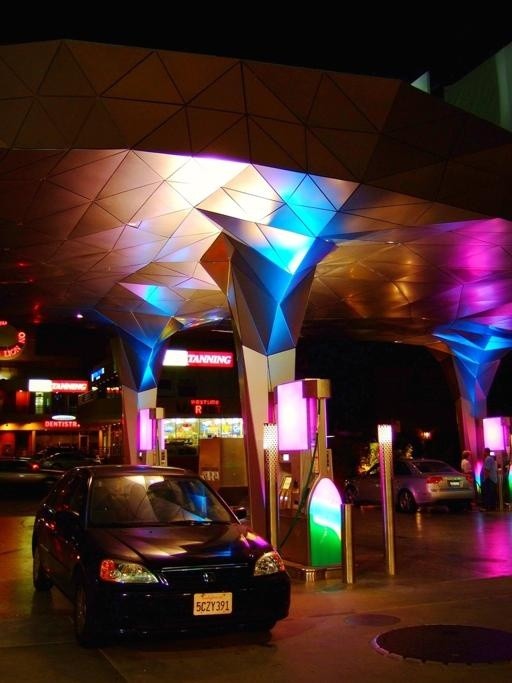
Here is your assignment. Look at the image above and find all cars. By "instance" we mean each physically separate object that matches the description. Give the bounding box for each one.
[0,445,89,509]
[342,456,475,513]
[33,459,291,643]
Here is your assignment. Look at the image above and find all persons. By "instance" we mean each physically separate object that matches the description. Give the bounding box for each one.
[460,449,478,511]
[482,446,498,512]
